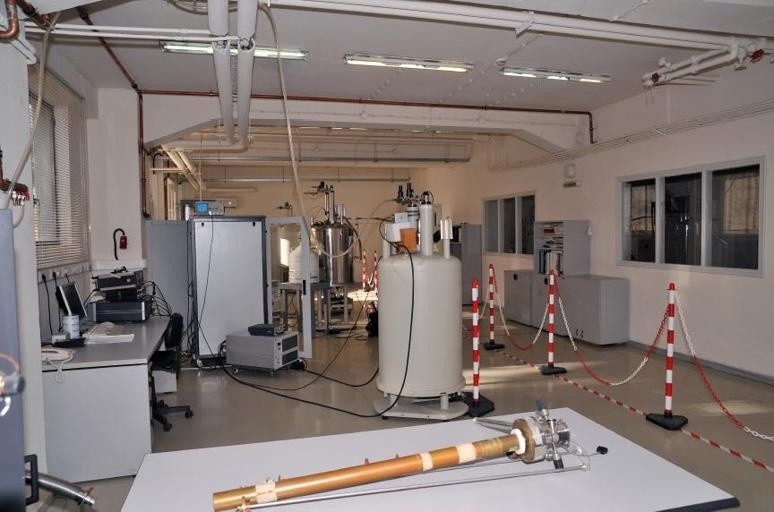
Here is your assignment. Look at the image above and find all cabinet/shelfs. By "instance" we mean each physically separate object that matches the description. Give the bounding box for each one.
[534,219,591,335]
[561,275,630,344]
[451,224,482,305]
[504,269,533,327]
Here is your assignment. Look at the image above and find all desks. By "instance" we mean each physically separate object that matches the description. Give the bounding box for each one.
[42,316,172,485]
[121,407,741,512]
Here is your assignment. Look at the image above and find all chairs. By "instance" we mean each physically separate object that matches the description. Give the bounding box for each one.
[150,314,192,431]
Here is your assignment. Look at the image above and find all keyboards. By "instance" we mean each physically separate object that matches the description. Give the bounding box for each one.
[82,325,113,339]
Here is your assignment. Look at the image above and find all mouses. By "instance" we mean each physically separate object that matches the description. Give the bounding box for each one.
[101,322,115,325]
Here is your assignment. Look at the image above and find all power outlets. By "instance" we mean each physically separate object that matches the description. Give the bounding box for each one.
[39,266,61,284]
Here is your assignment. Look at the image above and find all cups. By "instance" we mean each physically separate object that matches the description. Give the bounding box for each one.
[62,314,80,339]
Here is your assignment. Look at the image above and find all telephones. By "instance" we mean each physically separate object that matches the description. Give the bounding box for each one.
[42,346,75,382]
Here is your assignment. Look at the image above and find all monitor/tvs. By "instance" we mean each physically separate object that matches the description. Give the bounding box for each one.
[56,281,87,319]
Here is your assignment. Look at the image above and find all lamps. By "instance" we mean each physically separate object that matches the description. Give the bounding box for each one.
[343,53,475,73]
[160,39,309,61]
[500,67,611,87]
[111,228,127,274]
